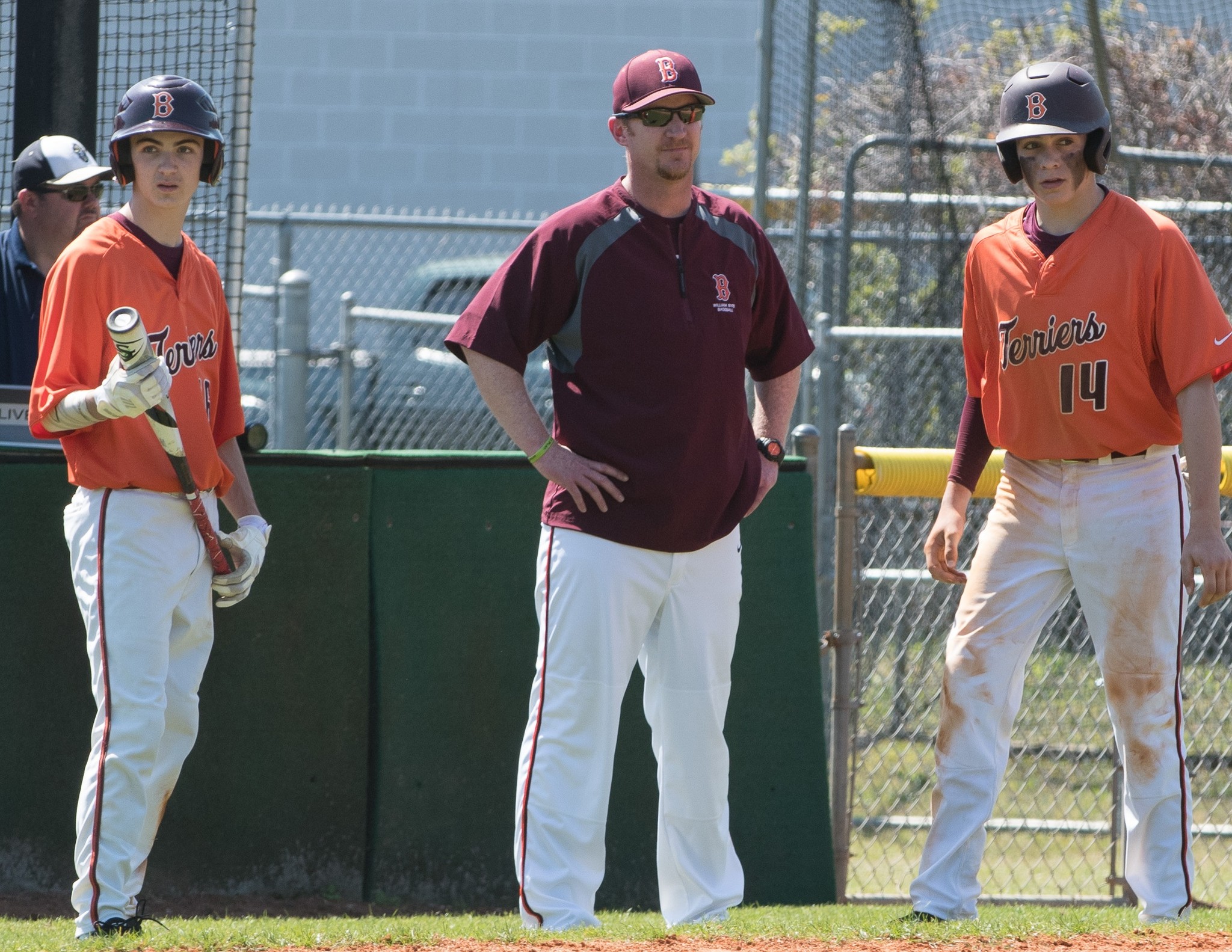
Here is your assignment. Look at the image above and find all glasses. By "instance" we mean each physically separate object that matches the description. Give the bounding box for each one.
[26,184,105,201]
[614,103,705,127]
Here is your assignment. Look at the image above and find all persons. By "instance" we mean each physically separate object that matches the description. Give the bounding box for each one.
[29,78,272,943]
[446,50,817,933]
[895,59,1232,927]
[0,134,269,448]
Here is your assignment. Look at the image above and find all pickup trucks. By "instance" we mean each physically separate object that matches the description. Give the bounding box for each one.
[243,257,558,455]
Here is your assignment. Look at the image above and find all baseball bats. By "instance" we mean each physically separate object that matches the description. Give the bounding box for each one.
[106,306,232,576]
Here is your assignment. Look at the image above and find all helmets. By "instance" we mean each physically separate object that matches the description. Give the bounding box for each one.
[995,61,1113,184]
[109,75,225,187]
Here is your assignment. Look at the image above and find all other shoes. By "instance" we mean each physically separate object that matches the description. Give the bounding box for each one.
[78,899,170,940]
[888,911,945,924]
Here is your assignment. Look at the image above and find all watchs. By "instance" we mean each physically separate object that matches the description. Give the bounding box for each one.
[754,435,786,463]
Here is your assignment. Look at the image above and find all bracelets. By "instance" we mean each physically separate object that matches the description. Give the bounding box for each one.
[527,435,554,464]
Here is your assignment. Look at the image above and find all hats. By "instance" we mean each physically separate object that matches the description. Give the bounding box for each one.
[13,134,113,195]
[613,49,715,119]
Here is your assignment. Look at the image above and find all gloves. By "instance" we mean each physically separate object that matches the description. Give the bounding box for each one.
[93,352,172,419]
[211,524,272,608]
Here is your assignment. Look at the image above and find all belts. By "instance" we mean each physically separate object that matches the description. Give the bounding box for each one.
[1061,449,1147,462]
